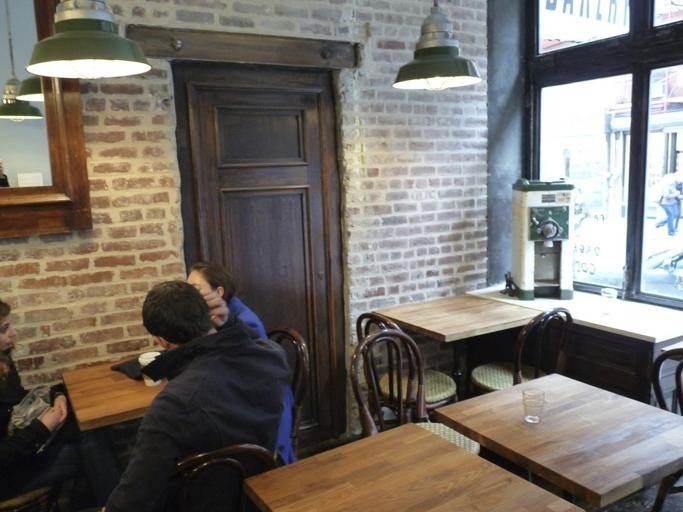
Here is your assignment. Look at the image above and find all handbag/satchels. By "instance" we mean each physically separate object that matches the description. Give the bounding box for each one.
[8,385,64,454]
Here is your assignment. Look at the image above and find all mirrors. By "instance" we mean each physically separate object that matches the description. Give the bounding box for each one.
[0,0,94,239]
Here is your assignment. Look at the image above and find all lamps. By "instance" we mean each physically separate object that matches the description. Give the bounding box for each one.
[25,1,152,79]
[15,76,45,103]
[0,1,42,121]
[392,0,481,91]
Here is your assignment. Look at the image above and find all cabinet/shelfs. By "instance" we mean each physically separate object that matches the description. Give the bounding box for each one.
[467,282,683,407]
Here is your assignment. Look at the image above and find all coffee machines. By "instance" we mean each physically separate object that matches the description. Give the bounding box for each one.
[500,178,575,300]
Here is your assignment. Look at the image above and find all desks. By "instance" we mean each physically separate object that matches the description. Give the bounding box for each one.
[63,353,168,432]
[435,373,683,507]
[244,424,587,512]
[374,294,544,401]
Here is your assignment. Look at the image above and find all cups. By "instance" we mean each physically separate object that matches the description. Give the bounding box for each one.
[138,351,162,387]
[522,387,545,423]
[601,287,618,314]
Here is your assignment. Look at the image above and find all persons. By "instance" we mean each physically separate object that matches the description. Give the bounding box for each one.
[97,280,289,512]
[0,300,116,512]
[0,159,10,187]
[186,261,299,478]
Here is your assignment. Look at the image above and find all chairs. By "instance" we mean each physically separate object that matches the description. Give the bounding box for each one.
[470,308,572,392]
[0,483,55,512]
[653,348,683,512]
[266,328,310,457]
[357,313,459,422]
[171,443,281,512]
[349,330,479,457]
